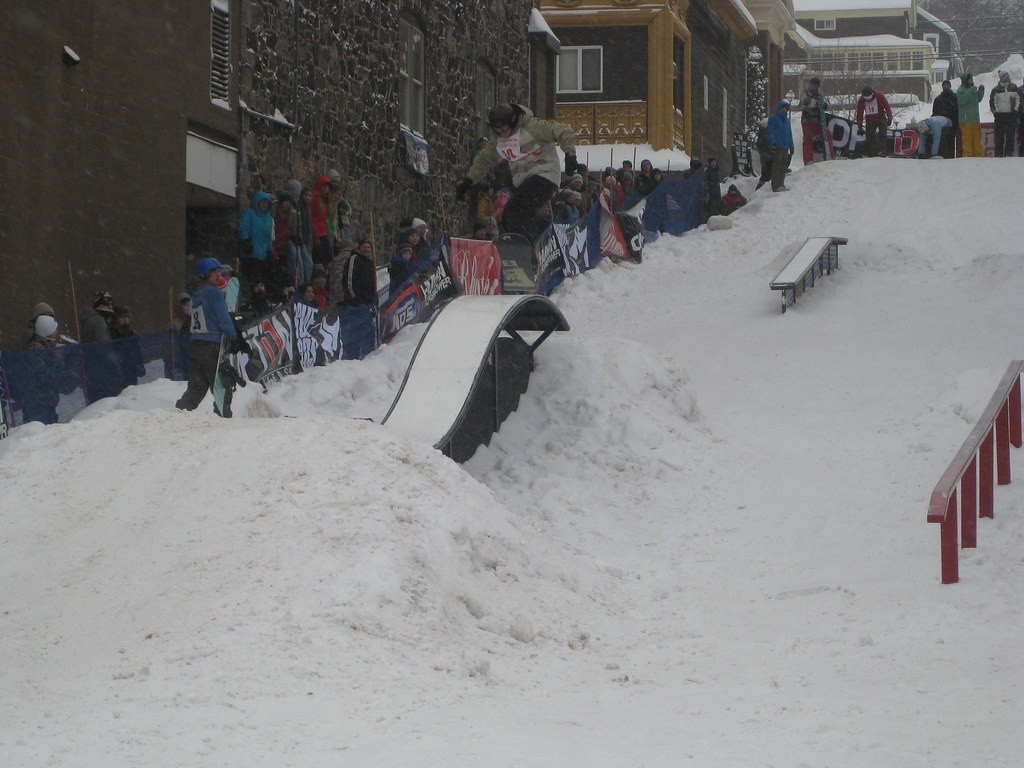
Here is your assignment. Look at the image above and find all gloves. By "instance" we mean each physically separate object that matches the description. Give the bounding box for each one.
[859,127,863,132]
[239,239,253,255]
[565,155,579,176]
[887,120,892,126]
[455,177,472,201]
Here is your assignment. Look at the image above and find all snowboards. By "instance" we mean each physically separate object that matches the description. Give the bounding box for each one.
[493,232,538,294]
[210,349,247,418]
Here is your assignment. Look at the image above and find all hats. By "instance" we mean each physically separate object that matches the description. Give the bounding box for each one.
[33,301,55,321]
[862,85,873,100]
[553,118,769,207]
[34,315,58,337]
[473,222,485,232]
[312,216,428,279]
[280,189,292,202]
[998,70,1010,80]
[942,79,951,88]
[114,303,131,318]
[94,290,113,307]
[918,119,930,134]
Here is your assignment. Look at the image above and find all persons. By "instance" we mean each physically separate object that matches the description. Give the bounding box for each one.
[172,169,428,323]
[957,73,984,157]
[756,113,780,191]
[916,115,954,159]
[469,137,596,259]
[575,157,747,241]
[767,101,794,192]
[176,257,237,418]
[1016,78,1024,157]
[990,70,1021,157]
[19,290,145,428]
[856,86,892,157]
[801,77,836,166]
[931,80,959,158]
[456,103,577,276]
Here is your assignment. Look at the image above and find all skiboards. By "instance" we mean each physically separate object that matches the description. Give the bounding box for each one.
[819,101,831,161]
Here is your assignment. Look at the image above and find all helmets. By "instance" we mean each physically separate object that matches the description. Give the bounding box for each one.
[488,103,516,126]
[200,272,227,288]
[195,257,221,277]
